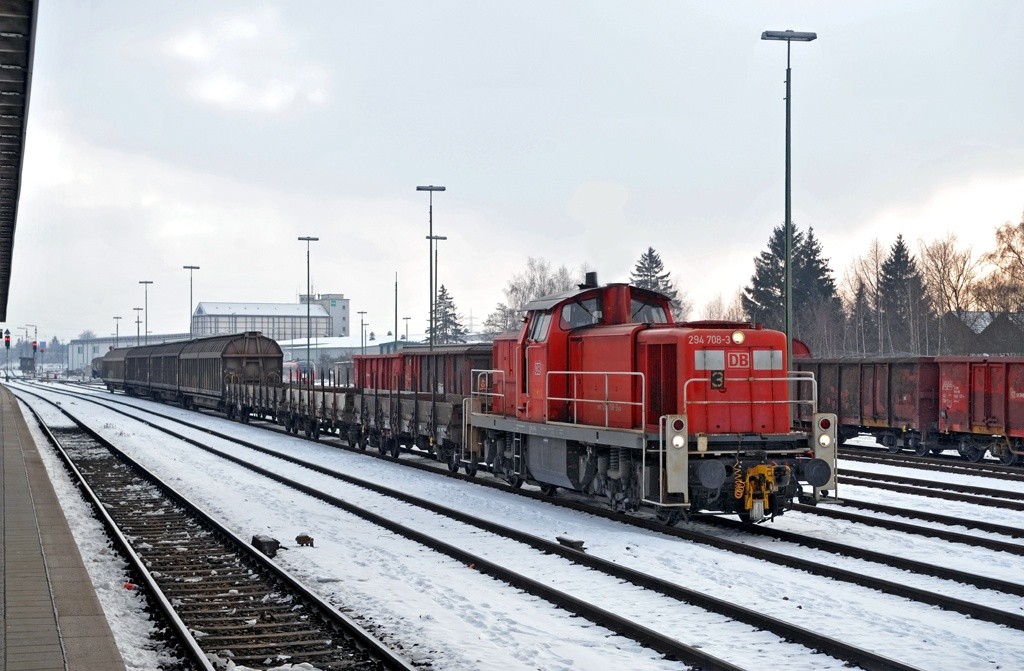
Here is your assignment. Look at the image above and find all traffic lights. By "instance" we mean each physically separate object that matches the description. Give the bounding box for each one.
[33,342,36,352]
[5,336,10,346]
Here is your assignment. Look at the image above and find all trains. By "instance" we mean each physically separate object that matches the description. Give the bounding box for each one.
[100,273,839,526]
[792,356,1024,468]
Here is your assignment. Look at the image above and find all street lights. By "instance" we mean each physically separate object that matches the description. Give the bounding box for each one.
[761,30,817,423]
[363,324,369,354]
[138,281,153,345]
[425,236,447,345]
[18,325,37,342]
[403,318,411,341]
[113,317,122,348]
[297,237,319,371]
[183,266,200,339]
[133,308,143,346]
[357,311,367,354]
[416,185,446,352]
